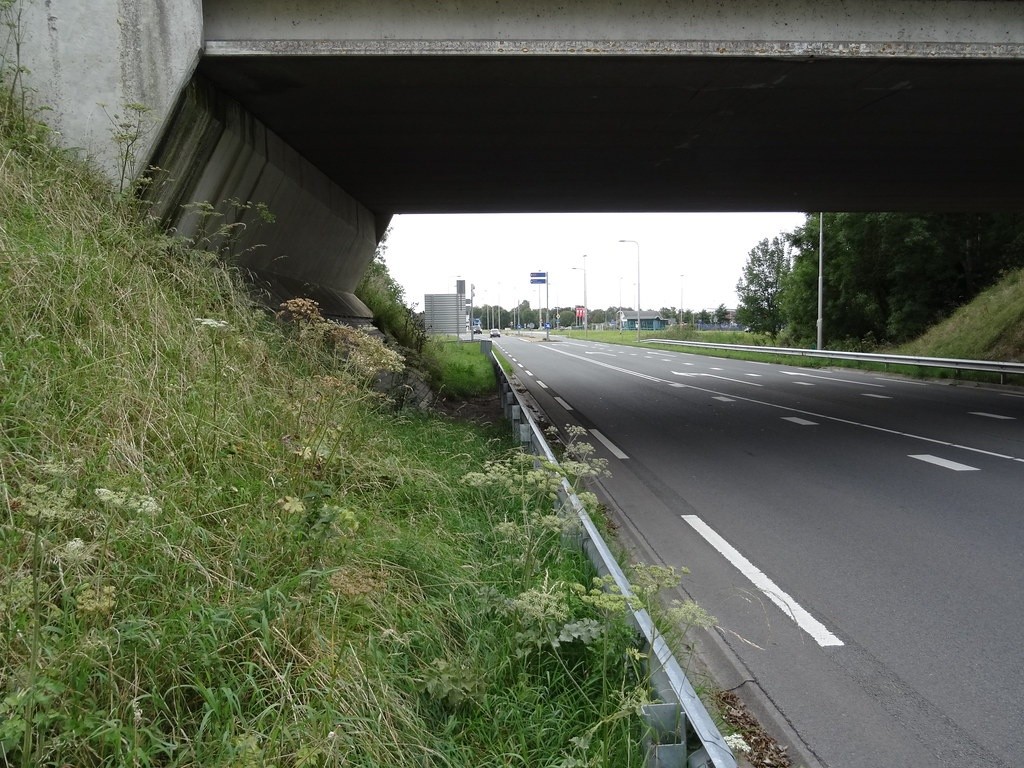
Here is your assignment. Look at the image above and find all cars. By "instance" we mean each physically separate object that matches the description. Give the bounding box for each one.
[473,326,483,334]
[489,329,501,338]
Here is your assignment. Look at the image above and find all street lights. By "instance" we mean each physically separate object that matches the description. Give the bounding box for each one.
[572,268,588,341]
[619,240,640,343]
[680,275,684,328]
[583,255,587,268]
